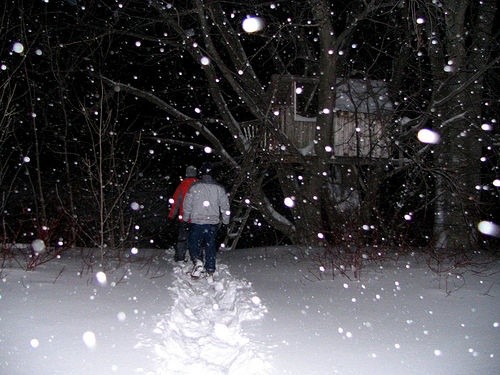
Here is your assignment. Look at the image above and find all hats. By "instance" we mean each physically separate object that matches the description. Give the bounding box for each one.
[185,166,197,177]
[201,163,212,174]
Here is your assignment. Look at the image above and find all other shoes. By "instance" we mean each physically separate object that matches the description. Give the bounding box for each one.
[177,258,188,263]
[207,272,213,278]
[192,262,203,278]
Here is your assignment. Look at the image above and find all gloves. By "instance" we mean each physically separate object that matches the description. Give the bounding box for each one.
[183,222,189,232]
[220,223,227,235]
[167,218,172,226]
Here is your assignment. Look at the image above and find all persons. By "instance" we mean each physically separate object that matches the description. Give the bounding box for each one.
[183,167,230,280]
[169,164,200,263]
